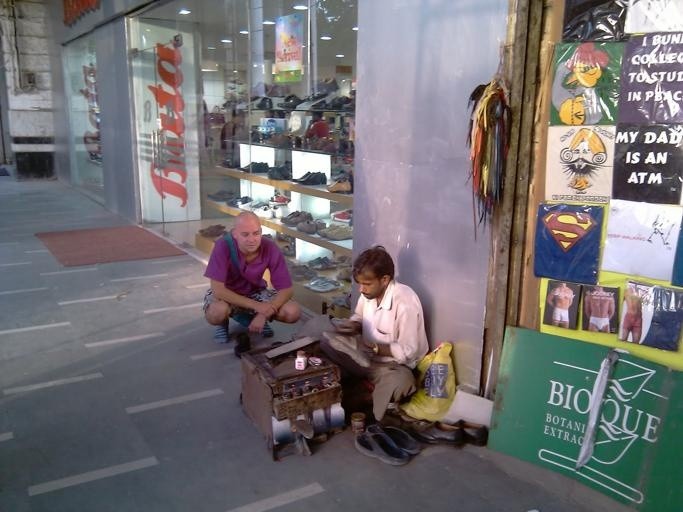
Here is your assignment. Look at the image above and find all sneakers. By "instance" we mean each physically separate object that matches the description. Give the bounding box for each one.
[324,332,371,369]
[198,190,352,310]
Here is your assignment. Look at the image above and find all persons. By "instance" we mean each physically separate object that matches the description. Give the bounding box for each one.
[584,287,616,333]
[306,111,329,137]
[620,284,651,343]
[201,210,301,343]
[320,245,429,421]
[547,282,575,329]
[219,108,248,150]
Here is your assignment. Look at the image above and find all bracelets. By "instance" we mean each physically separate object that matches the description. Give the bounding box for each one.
[367,343,379,355]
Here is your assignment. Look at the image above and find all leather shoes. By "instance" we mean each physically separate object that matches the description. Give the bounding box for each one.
[408,420,465,447]
[220,78,353,194]
[452,419,489,446]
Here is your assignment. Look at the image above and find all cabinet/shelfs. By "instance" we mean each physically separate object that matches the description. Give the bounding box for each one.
[194,107,354,316]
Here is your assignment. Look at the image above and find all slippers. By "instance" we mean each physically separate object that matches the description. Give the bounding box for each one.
[354,431,410,466]
[366,424,421,455]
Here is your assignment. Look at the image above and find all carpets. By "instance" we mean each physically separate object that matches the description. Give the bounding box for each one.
[34,225,188,267]
